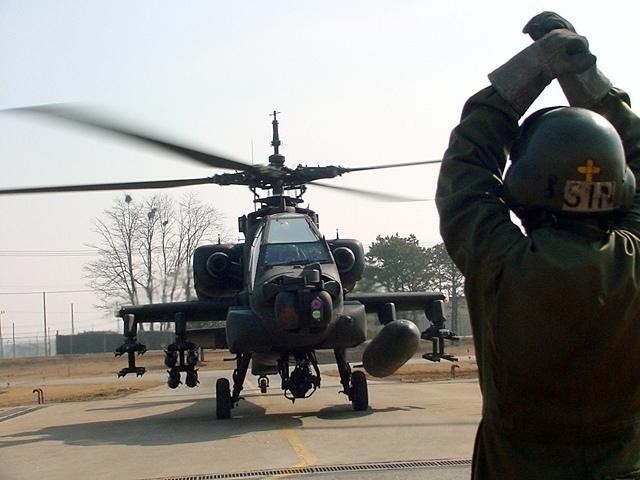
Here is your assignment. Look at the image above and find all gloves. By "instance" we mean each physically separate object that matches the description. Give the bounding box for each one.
[487,30,594,116]
[520,9,612,110]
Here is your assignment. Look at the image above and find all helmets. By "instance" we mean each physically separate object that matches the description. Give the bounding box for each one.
[503,106,638,217]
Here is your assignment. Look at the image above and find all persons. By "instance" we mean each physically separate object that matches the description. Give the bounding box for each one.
[435,10,640,480]
